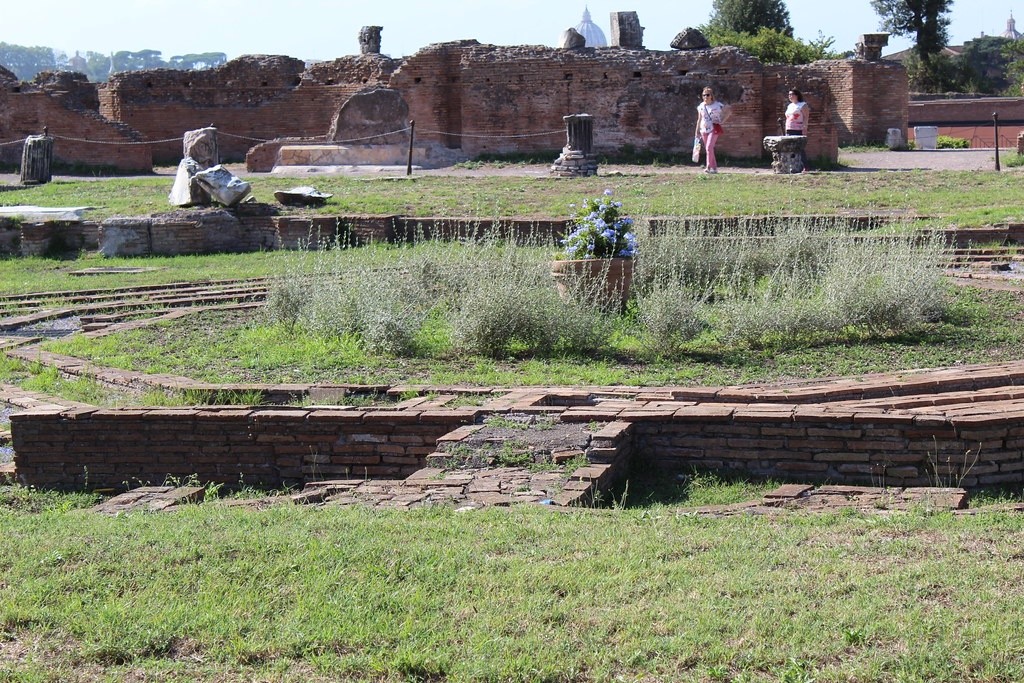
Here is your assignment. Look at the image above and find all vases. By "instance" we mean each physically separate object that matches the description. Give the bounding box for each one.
[548,259,634,314]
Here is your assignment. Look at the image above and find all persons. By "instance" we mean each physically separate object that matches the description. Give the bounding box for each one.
[694,86,732,174]
[784,88,811,173]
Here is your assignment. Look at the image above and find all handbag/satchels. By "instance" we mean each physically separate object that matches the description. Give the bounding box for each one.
[712,123,724,134]
[692,139,701,163]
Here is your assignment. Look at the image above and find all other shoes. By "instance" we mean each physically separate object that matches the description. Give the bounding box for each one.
[705,168,718,173]
[803,166,808,171]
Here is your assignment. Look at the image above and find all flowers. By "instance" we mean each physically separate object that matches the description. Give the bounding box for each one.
[554,188,635,258]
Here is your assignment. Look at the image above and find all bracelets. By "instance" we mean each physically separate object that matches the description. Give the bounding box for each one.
[803,125,808,129]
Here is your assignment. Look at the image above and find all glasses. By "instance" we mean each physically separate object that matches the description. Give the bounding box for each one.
[702,94,709,96]
